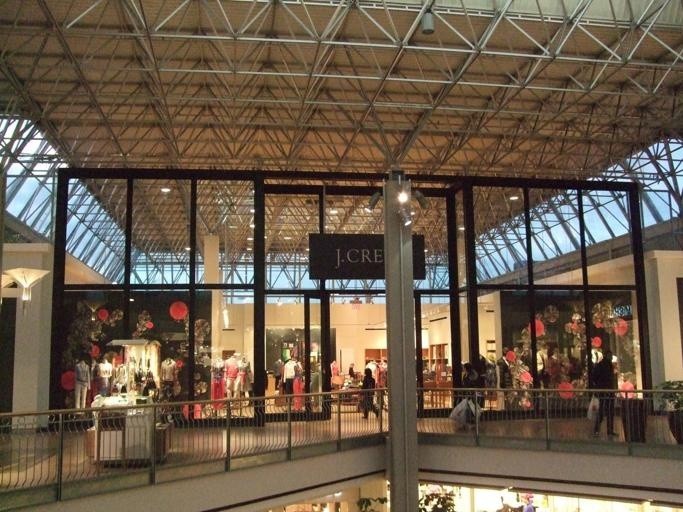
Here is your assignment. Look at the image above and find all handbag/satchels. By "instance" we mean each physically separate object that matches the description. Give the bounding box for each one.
[586,392,600,421]
[449,396,482,423]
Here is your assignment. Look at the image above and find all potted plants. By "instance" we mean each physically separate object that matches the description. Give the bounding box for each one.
[652,380,683,445]
[621,397,649,444]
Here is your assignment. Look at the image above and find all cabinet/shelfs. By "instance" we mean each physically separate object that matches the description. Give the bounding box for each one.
[86,338,175,466]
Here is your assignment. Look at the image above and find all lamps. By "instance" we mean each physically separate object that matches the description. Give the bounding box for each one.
[364,169,432,227]
[0,267,50,304]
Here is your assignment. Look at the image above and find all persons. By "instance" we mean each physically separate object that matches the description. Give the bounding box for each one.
[223,355,238,408]
[283,359,297,402]
[360,367,379,420]
[348,362,358,379]
[496,346,586,410]
[589,350,619,439]
[236,357,252,406]
[273,359,284,390]
[211,357,223,399]
[96,358,113,395]
[461,363,481,425]
[72,359,90,417]
[160,357,177,382]
[483,353,499,413]
[330,360,339,377]
[292,365,303,411]
